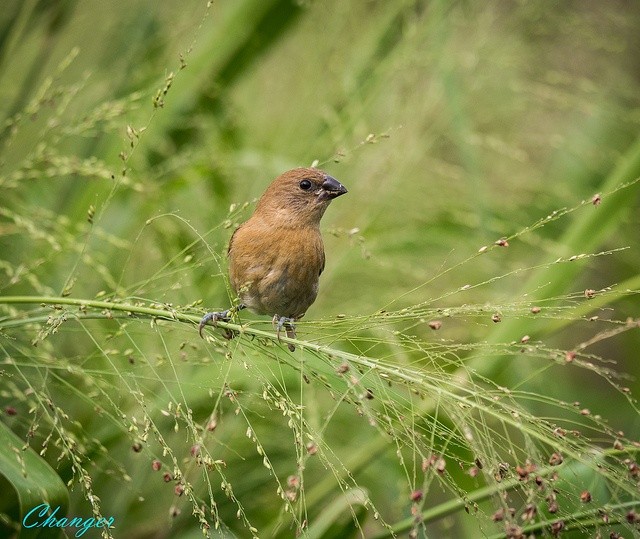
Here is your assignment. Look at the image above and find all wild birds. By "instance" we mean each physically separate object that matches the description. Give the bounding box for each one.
[199,168,349,352]
